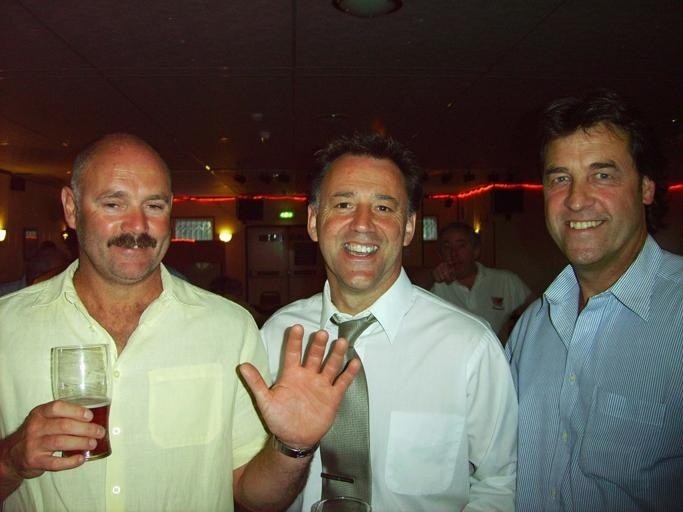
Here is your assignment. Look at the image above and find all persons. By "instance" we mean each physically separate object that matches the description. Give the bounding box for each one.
[0,132,362,511]
[420,222,537,354]
[0,234,67,297]
[501,91,682,512]
[257,132,517,511]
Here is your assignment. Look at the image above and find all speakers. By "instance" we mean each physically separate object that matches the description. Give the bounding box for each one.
[495,190,523,210]
[239,200,263,219]
[11,177,25,191]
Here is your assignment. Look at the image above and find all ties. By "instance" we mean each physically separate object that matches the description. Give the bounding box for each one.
[318,312,380,509]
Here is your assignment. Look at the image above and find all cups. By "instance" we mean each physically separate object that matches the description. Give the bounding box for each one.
[49,344,111,465]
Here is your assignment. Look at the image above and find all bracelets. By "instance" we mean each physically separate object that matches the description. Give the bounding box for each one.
[268,430,319,460]
